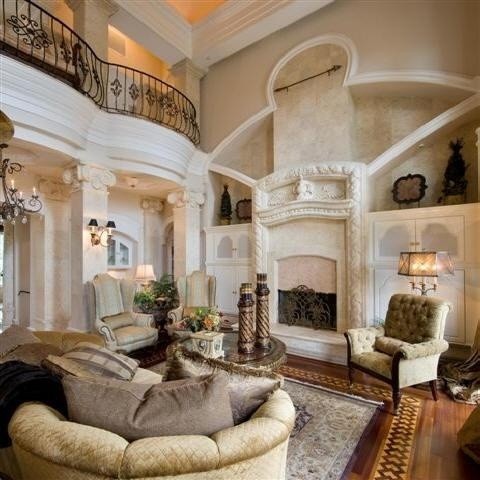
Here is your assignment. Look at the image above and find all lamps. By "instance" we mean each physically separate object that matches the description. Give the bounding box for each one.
[86,216,116,251]
[133,263,157,292]
[1,110,46,235]
[395,250,439,297]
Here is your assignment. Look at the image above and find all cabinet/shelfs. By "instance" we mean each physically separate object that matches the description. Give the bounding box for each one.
[363,203,480,265]
[203,223,256,265]
[106,238,132,269]
[205,264,257,316]
[362,264,479,350]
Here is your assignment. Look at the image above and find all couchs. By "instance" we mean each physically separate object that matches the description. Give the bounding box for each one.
[0,323,297,480]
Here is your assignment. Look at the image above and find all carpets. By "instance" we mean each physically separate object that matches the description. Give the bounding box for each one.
[132,356,389,480]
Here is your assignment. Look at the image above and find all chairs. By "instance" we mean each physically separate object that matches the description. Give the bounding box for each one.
[344,291,451,418]
[91,271,161,354]
[164,269,218,339]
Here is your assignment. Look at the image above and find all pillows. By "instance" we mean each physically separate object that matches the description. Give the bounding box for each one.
[182,307,207,319]
[373,335,410,357]
[37,331,287,440]
[102,311,135,330]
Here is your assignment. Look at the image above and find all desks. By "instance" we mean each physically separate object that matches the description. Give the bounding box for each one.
[138,303,179,331]
